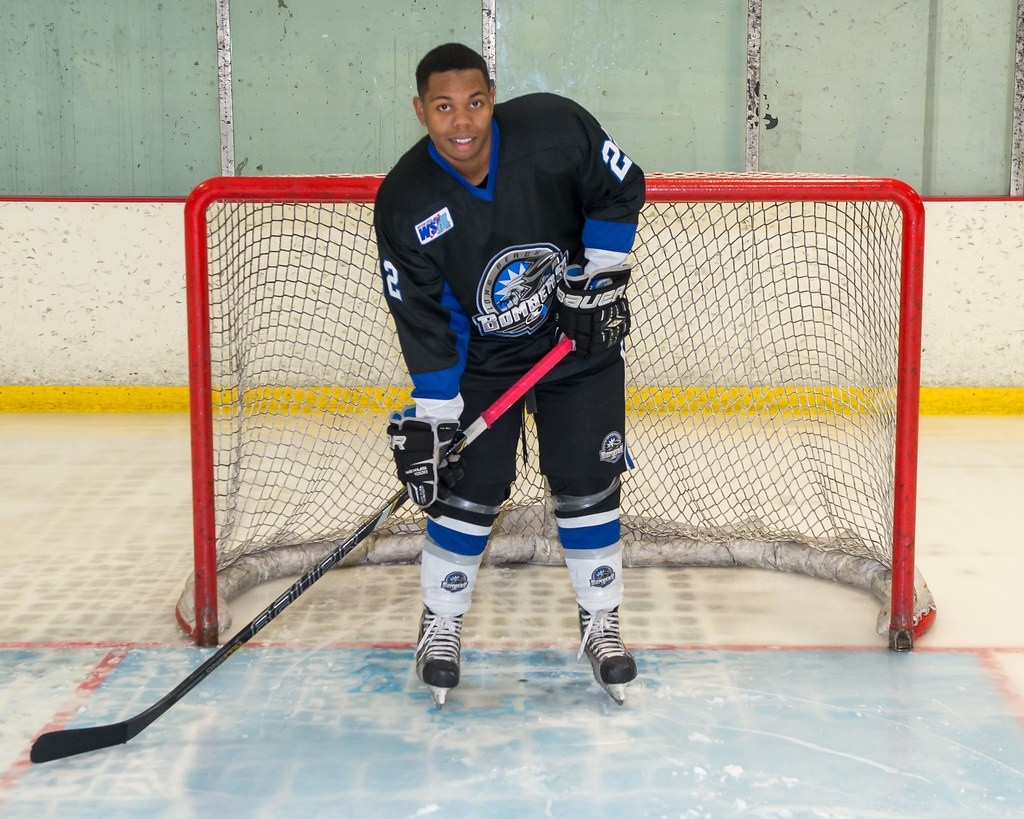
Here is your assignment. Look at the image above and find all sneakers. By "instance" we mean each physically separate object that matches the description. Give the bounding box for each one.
[414,603,464,710]
[576,602,637,706]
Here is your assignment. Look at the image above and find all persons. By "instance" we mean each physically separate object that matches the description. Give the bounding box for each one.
[374,44,646,710]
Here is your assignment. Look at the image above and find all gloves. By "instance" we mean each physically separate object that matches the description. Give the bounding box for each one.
[551,263,632,360]
[386,404,466,519]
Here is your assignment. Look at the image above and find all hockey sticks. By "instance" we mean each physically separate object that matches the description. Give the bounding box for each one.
[31,333,574,763]
